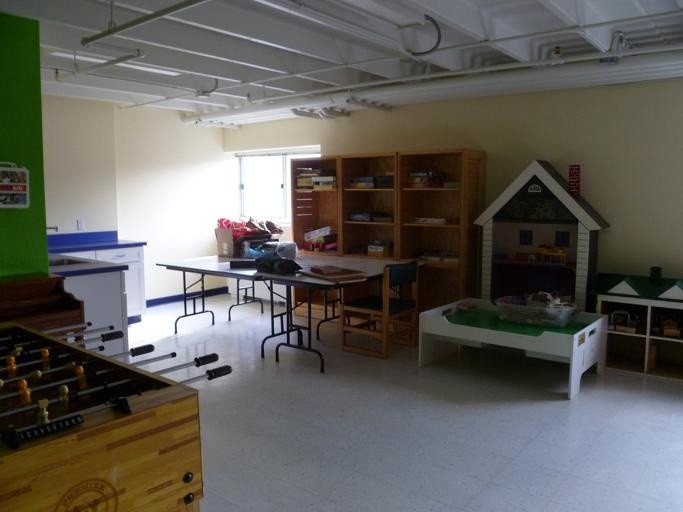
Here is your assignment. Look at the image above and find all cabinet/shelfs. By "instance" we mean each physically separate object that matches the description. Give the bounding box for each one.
[289,149,486,347]
[596,295,683,387]
[0,247,146,358]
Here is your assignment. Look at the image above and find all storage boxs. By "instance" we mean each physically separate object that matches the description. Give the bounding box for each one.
[215,228,272,256]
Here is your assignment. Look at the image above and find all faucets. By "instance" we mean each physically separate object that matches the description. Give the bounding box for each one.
[46,225,59,233]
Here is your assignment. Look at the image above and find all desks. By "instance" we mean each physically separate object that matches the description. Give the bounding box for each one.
[157,256,415,373]
[420,296,606,400]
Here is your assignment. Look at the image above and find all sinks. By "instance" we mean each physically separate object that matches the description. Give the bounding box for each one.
[48,257,92,264]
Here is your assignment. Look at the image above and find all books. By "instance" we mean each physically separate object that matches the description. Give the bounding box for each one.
[229,258,256,268]
[298,261,369,279]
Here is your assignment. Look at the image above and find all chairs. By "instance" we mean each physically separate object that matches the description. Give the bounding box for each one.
[337,260,419,360]
